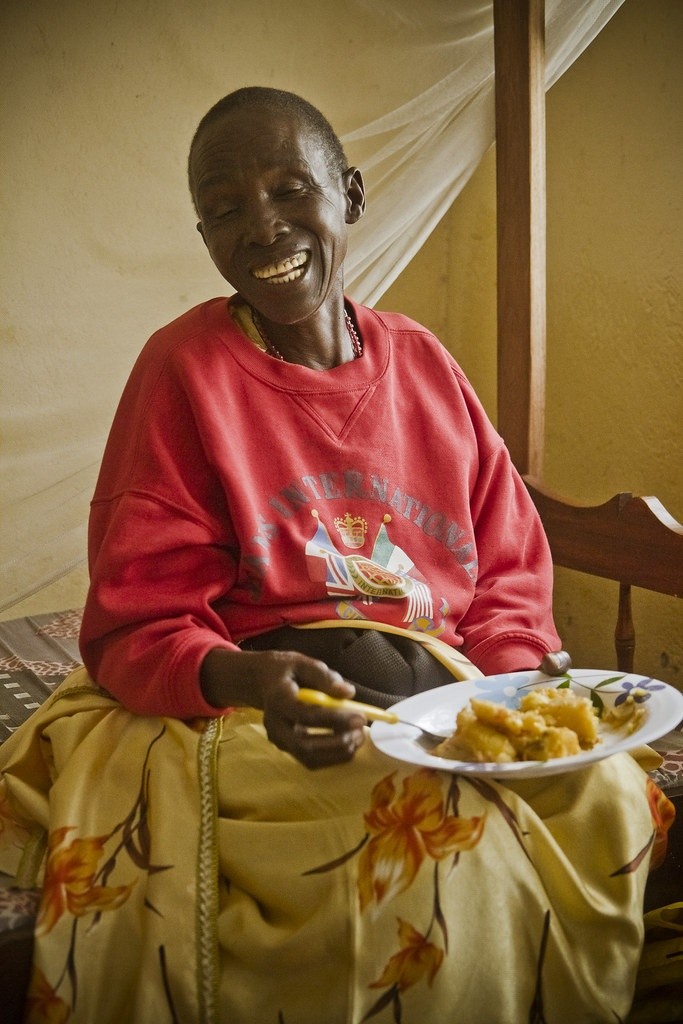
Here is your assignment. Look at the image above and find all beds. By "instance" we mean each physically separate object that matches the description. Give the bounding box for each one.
[0,470,683,953]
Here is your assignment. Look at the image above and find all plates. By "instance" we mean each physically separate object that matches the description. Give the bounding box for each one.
[369,668,683,779]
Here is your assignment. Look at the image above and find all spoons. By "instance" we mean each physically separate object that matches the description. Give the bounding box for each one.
[296,687,456,742]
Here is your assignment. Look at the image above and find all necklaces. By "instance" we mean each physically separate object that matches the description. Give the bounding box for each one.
[250,307,363,364]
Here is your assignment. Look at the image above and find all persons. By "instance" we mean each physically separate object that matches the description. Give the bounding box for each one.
[80,86,673,1024]
